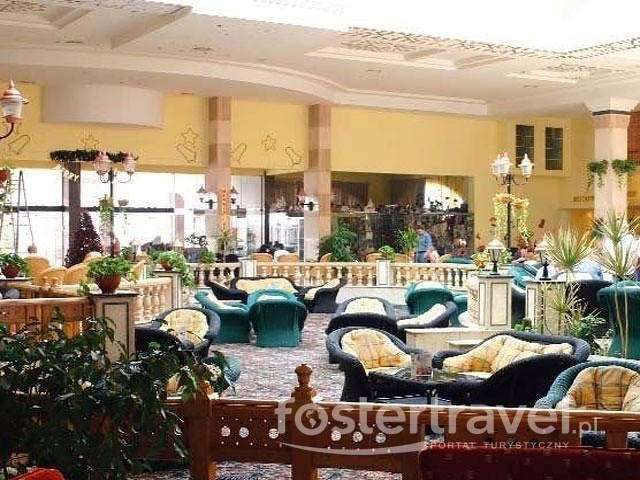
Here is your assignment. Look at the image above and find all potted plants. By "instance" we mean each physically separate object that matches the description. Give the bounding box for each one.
[0,253,32,279]
[585,159,637,192]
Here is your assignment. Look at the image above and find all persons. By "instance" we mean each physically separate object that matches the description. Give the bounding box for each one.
[0,281,21,299]
[413,224,434,263]
[102,230,121,256]
[511,247,528,264]
[273,240,286,252]
[140,236,166,254]
[256,241,273,253]
[129,238,142,255]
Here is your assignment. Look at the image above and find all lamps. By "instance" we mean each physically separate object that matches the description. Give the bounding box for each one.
[295,187,314,211]
[196,184,239,210]
[534,236,556,280]
[0,77,29,141]
[486,239,506,276]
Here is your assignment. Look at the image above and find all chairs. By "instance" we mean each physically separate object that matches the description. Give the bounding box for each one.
[12,252,640,480]
